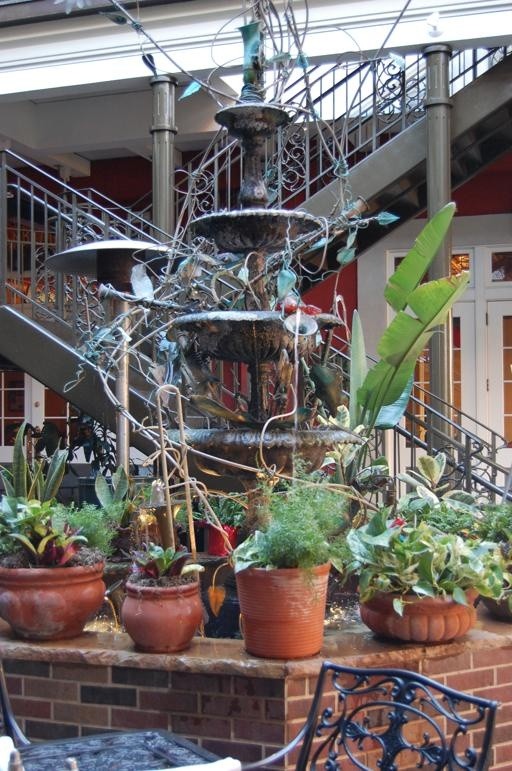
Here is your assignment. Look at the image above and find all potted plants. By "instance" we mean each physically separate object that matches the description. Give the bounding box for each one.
[181,490,251,561]
[115,537,209,653]
[3,492,110,644]
[347,448,508,645]
[233,451,355,660]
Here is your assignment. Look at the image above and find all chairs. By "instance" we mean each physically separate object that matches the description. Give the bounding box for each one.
[0,662,33,745]
[241,656,499,771]
